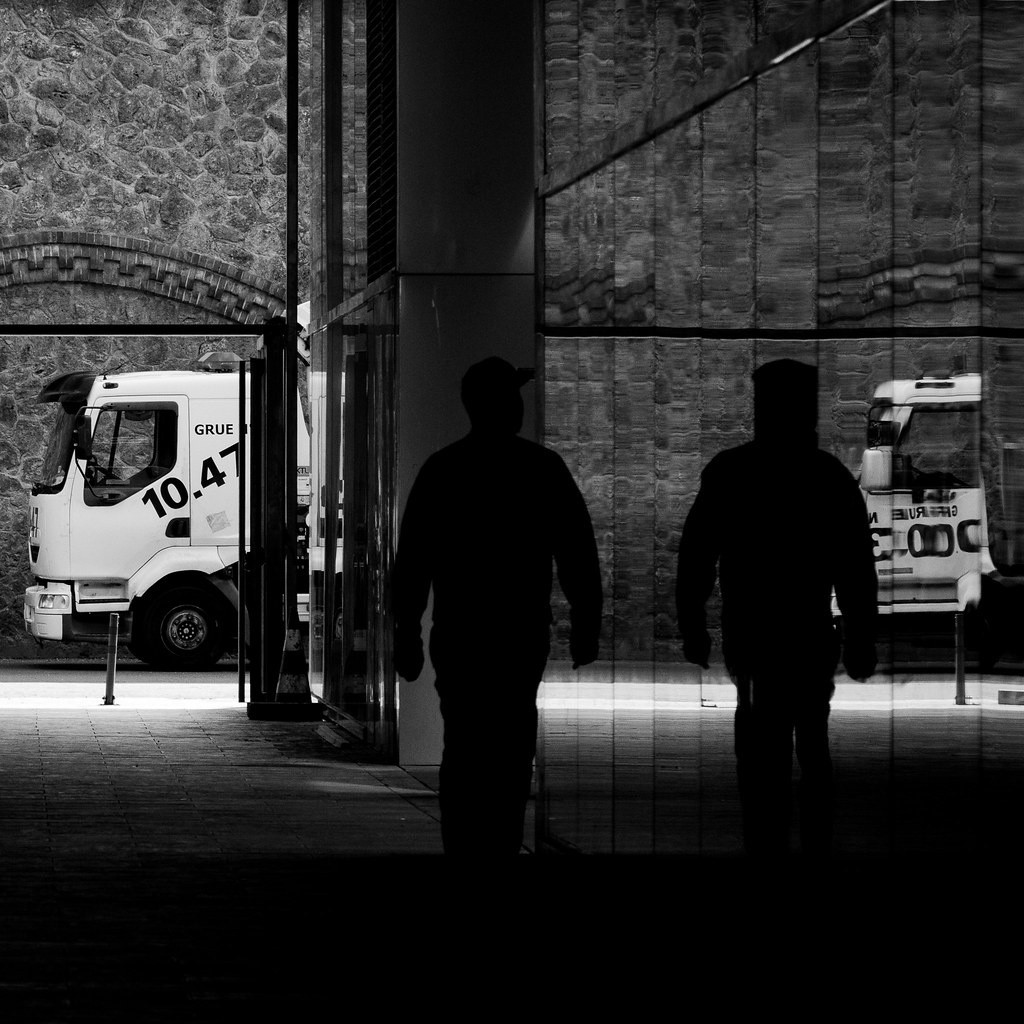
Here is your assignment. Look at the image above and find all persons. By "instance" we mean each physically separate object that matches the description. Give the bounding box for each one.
[384,357,603,853]
[675,360,876,854]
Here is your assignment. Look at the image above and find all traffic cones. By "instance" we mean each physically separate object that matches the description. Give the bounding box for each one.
[274,611,312,703]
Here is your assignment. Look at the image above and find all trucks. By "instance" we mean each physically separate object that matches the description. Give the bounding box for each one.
[23,301,343,672]
[830,369,997,678]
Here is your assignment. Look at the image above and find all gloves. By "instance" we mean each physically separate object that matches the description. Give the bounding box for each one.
[683,630,712,671]
[569,626,601,669]
[391,625,424,683]
[843,640,878,681]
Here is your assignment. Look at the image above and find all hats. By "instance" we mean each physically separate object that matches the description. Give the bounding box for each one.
[459,358,535,409]
